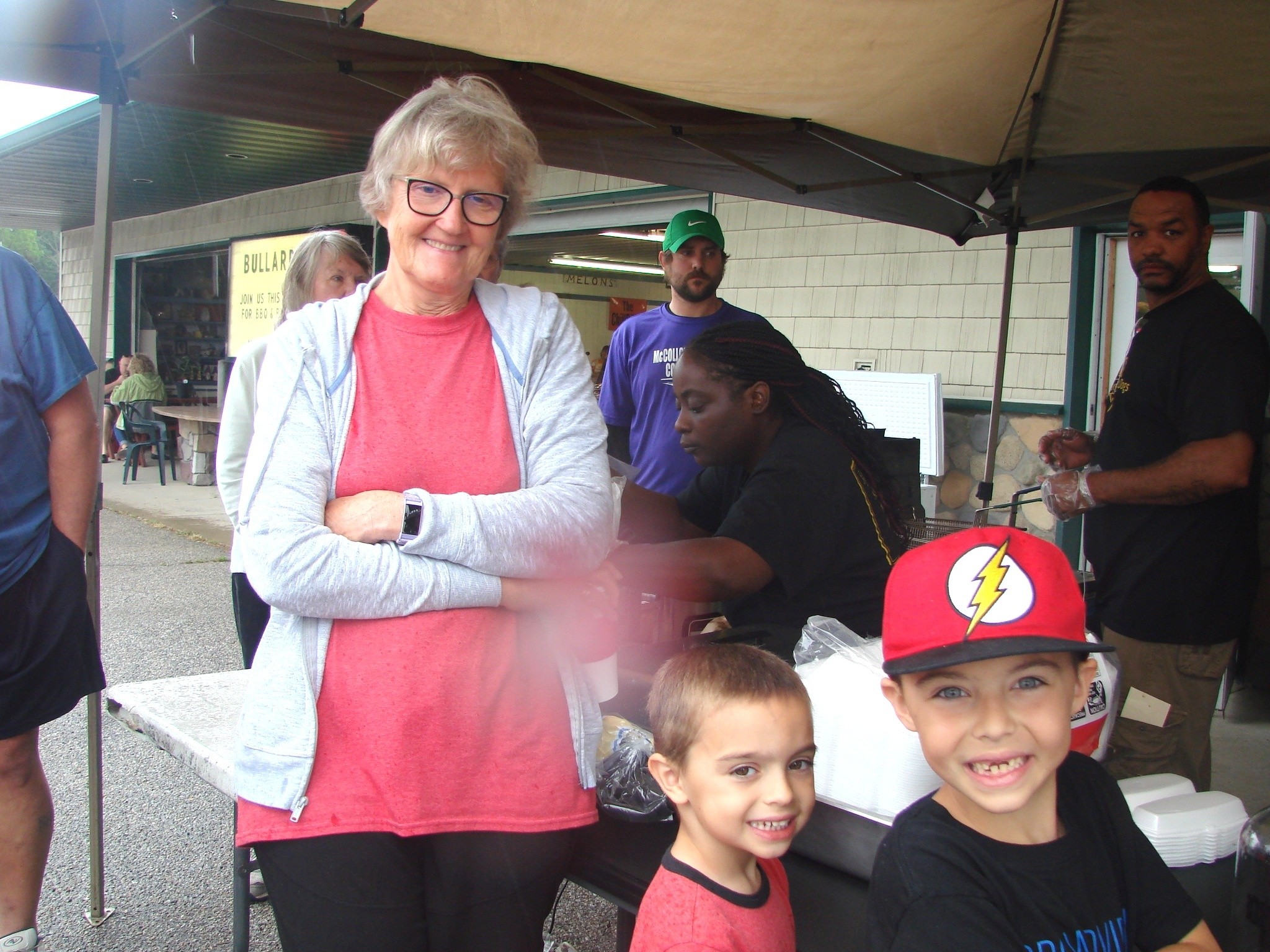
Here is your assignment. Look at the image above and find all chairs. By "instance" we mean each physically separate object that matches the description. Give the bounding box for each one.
[119,401,178,485]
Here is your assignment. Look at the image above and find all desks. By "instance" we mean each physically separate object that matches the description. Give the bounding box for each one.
[107,669,262,952]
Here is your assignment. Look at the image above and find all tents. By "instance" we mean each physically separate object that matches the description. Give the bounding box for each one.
[1,1,1270,923]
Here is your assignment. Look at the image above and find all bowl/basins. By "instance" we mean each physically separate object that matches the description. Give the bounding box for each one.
[1118,773,1251,868]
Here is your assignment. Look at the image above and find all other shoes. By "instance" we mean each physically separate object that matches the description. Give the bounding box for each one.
[123,456,132,467]
[117,446,129,459]
[152,452,169,460]
[0,928,45,952]
[100,454,108,463]
[250,868,268,902]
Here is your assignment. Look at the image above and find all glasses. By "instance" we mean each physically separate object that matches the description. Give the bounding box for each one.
[393,174,511,227]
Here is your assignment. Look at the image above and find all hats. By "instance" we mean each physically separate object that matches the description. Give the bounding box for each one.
[663,209,724,252]
[881,527,1114,675]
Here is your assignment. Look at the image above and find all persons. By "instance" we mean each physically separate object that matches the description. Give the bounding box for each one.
[626,642,818,951]
[476,234,507,283]
[598,209,765,498]
[0,242,108,951]
[870,527,1223,951]
[111,353,172,460]
[1036,176,1270,793]
[101,354,132,464]
[235,82,615,952]
[214,231,374,902]
[105,357,114,369]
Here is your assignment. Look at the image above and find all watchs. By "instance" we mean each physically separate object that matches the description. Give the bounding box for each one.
[395,492,423,545]
[616,341,916,642]
